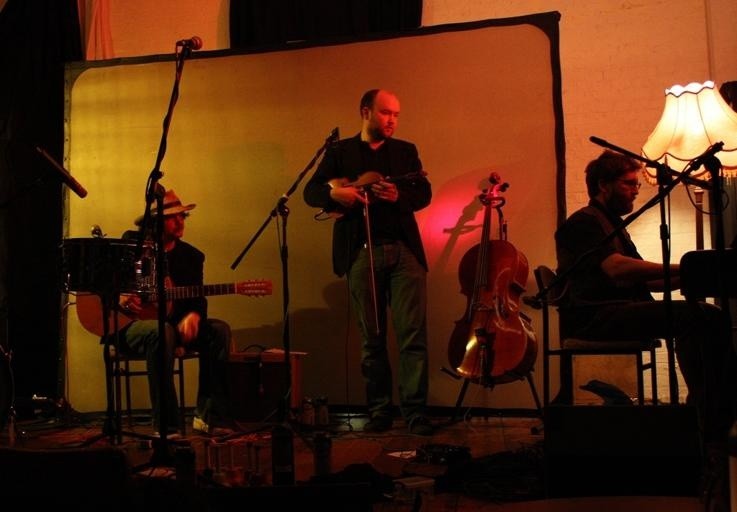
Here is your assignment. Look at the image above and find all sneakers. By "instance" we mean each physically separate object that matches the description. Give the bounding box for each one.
[161,427,182,439]
[193,415,235,436]
[364,414,391,432]
[409,417,434,435]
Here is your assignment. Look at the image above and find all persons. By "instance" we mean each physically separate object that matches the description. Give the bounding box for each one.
[113,190,240,439]
[555,151,721,407]
[303,88,436,435]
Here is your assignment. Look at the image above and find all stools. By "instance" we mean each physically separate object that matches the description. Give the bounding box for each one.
[104,345,186,445]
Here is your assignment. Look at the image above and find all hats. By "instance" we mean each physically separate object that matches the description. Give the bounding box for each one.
[135,189,196,226]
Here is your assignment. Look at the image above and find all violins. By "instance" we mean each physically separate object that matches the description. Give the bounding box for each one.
[322,171,428,219]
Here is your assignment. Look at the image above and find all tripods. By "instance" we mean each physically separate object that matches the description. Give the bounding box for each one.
[449,204,543,431]
[75,280,164,451]
[221,135,330,451]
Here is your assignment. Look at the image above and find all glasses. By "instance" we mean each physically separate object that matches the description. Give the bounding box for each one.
[624,179,642,190]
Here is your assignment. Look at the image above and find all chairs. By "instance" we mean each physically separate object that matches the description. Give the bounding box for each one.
[533,264,662,451]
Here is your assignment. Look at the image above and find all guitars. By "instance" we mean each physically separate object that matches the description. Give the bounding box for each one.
[75,272,274,337]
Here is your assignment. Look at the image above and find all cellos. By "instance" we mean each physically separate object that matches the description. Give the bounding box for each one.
[447,172,538,390]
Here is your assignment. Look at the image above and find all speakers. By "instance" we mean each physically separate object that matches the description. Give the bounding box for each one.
[545,405,705,499]
[0,448,137,487]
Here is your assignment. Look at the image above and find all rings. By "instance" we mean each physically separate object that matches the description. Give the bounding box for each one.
[126,306,128,309]
[129,302,134,306]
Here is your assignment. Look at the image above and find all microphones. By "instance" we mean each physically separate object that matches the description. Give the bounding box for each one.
[257,362,265,395]
[179,36,203,50]
[34,143,87,198]
[330,128,339,151]
